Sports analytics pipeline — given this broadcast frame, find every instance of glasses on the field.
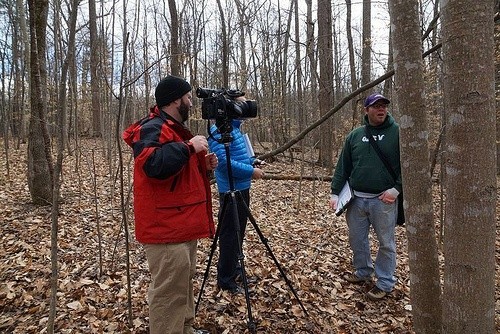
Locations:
[369,104,387,108]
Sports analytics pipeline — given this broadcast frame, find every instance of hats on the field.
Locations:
[364,93,390,108]
[155,75,191,107]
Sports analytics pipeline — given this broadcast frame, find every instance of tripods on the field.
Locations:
[195,134,310,334]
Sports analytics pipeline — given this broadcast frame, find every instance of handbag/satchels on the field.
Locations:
[397,193,405,226]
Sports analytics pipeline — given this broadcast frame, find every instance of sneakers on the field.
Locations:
[343,274,372,283]
[367,286,387,300]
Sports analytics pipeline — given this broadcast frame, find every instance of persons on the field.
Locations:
[209,88,265,296]
[123,75,218,334]
[330,94,405,300]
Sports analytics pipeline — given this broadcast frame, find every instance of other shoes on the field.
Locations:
[217,273,243,296]
